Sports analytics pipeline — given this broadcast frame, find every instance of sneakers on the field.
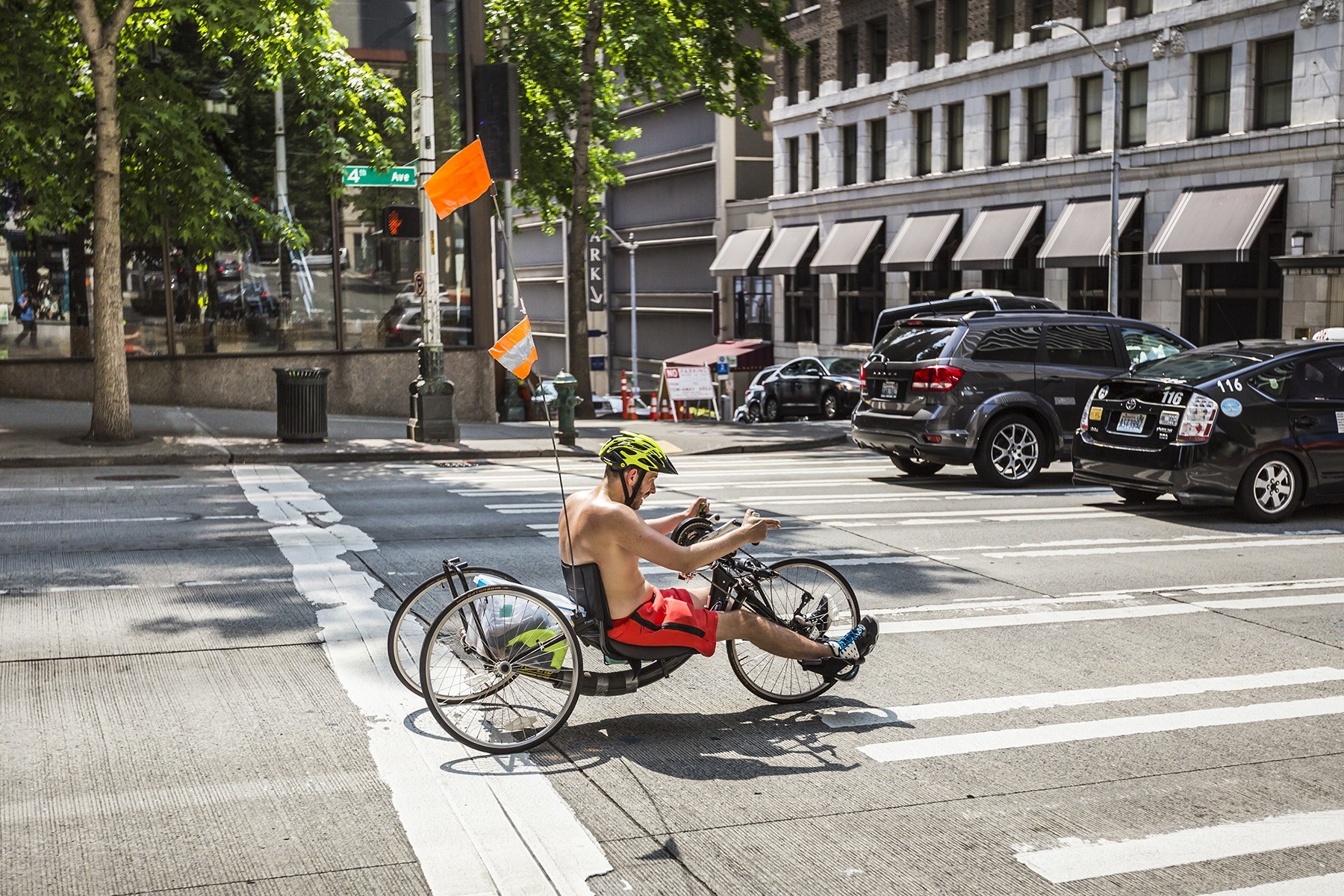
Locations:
[806,594,833,641]
[826,614,879,675]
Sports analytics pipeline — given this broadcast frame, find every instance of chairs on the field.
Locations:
[562,559,632,662]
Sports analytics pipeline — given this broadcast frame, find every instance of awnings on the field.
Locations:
[879,212,960,271]
[951,205,1044,270]
[1148,184,1285,266]
[757,225,819,275]
[1035,194,1143,268]
[810,219,884,275]
[709,228,772,276]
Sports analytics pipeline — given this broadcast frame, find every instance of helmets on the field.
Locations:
[599,431,678,474]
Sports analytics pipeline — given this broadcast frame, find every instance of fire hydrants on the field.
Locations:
[550,369,584,446]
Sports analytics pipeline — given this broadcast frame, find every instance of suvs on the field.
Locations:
[871,287,1071,352]
[845,310,1241,490]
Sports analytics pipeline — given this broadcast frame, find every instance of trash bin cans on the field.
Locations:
[271,366,332,441]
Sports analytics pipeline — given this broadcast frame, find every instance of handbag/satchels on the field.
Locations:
[11,297,22,318]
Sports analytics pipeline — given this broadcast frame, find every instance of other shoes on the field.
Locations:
[30,346,38,349]
[15,341,20,347]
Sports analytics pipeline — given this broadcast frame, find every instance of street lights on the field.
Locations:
[603,224,640,396]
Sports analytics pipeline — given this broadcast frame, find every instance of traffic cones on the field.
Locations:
[647,391,660,420]
[661,398,670,420]
[627,392,639,420]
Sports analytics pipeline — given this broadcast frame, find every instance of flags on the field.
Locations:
[422,138,492,220]
[487,313,540,381]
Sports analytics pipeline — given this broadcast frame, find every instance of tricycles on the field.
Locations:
[386,496,863,756]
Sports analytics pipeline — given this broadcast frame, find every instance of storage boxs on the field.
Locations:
[465,575,586,673]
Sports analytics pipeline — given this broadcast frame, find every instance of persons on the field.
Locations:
[558,429,880,682]
[1135,335,1160,363]
[14,288,40,348]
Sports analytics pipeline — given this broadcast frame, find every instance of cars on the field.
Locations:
[130,255,280,330]
[531,378,651,419]
[291,246,349,272]
[1069,336,1344,524]
[374,302,475,347]
[730,354,865,424]
[393,282,451,308]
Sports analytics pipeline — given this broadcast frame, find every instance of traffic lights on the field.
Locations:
[382,205,421,241]
